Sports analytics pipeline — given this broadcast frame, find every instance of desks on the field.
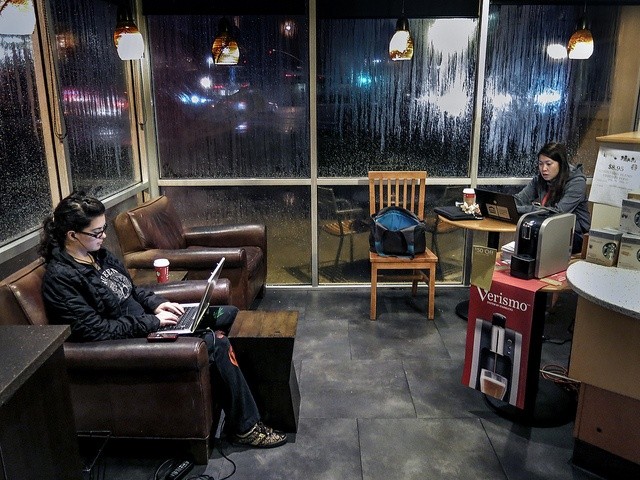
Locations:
[565,261,639,479]
[470,253,578,428]
[0,324,81,480]
[438,207,517,321]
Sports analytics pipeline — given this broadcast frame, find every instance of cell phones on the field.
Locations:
[149,333,179,342]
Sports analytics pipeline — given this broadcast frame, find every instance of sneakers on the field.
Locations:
[230,423,289,449]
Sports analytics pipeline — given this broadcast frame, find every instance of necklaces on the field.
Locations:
[73,253,94,264]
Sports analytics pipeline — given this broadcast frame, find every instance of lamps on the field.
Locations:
[113,8,145,60]
[389,1,414,61]
[212,7,239,65]
[568,0,595,60]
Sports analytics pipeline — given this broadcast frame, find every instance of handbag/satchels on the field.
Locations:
[369,206,426,259]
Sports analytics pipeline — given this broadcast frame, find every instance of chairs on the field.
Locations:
[317,186,369,271]
[368,171,439,320]
[424,187,469,282]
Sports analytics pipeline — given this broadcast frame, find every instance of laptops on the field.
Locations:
[433,204,483,221]
[150,255,227,336]
[475,188,522,224]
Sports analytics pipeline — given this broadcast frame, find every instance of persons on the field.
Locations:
[36,194,289,449]
[512,143,590,255]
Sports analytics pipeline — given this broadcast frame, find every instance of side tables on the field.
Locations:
[128,268,188,284]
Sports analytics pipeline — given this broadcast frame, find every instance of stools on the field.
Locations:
[227,310,302,434]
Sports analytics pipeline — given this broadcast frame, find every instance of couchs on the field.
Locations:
[0,257,232,430]
[112,194,267,311]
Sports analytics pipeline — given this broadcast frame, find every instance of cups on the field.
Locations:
[462,188,475,205]
[153,258,170,282]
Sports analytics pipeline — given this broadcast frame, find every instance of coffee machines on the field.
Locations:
[468,312,523,407]
[509,201,577,281]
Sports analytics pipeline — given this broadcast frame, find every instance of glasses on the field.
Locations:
[77,224,108,238]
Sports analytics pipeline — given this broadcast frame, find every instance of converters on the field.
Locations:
[165,458,193,479]
